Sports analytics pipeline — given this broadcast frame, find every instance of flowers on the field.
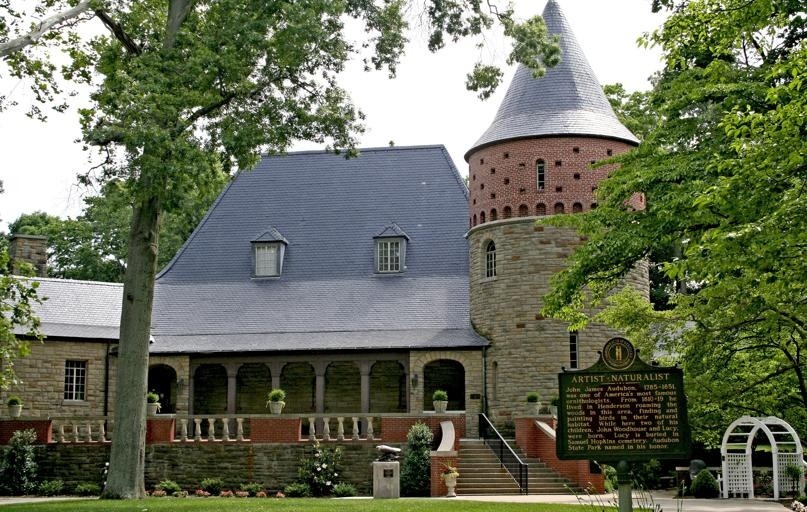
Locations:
[439,458,460,474]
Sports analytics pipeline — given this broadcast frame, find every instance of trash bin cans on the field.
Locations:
[372,461,400,499]
[675,467,690,490]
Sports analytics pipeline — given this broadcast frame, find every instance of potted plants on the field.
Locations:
[265,389,285,414]
[432,390,448,413]
[146,392,161,417]
[5,396,23,419]
[525,391,558,416]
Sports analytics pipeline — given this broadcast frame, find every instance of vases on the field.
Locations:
[443,473,458,497]
